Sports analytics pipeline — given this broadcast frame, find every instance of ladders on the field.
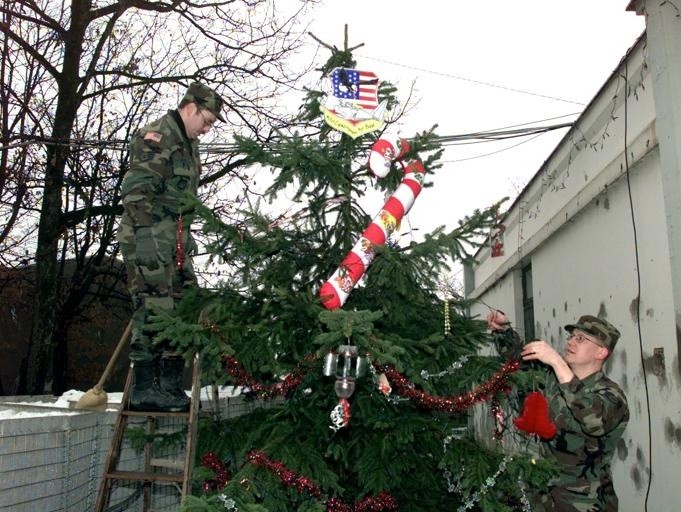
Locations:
[94,353,201,512]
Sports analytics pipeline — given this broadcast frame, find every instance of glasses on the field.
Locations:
[566,333,604,348]
[199,110,213,129]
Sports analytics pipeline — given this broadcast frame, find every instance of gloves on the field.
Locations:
[135,227,166,271]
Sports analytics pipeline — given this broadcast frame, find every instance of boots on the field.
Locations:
[128,355,202,413]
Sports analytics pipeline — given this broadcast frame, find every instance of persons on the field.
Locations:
[115,81,227,414]
[486,308,630,511]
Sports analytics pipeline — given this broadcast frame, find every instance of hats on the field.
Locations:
[564,315,621,352]
[184,81,226,123]
